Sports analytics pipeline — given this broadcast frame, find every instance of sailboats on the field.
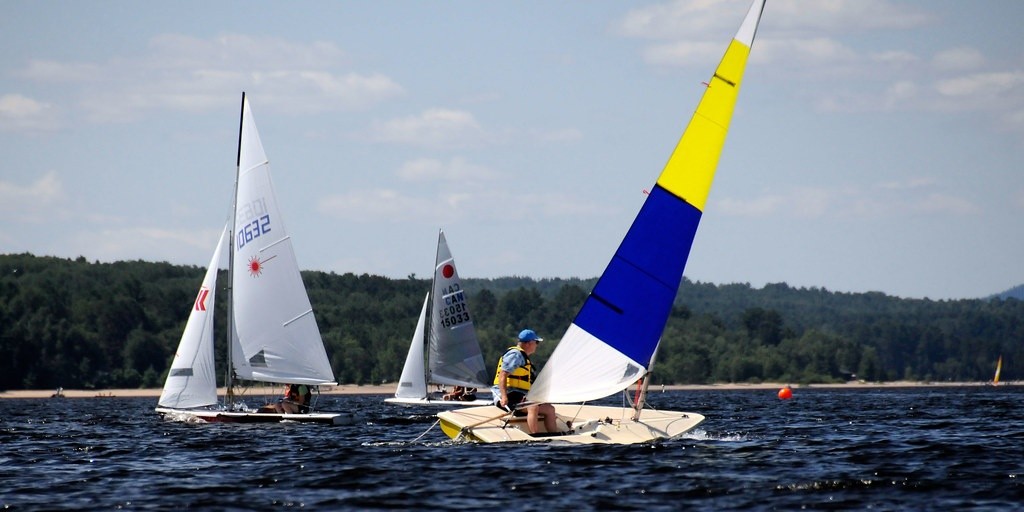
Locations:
[380,228,495,409]
[437,1,766,444]
[154,92,353,428]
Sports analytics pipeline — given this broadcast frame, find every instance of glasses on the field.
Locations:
[531,341,539,344]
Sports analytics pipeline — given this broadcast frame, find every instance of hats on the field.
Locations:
[518,329,543,342]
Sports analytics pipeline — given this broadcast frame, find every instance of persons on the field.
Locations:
[275,384,311,413]
[492,330,556,433]
[443,386,477,401]
